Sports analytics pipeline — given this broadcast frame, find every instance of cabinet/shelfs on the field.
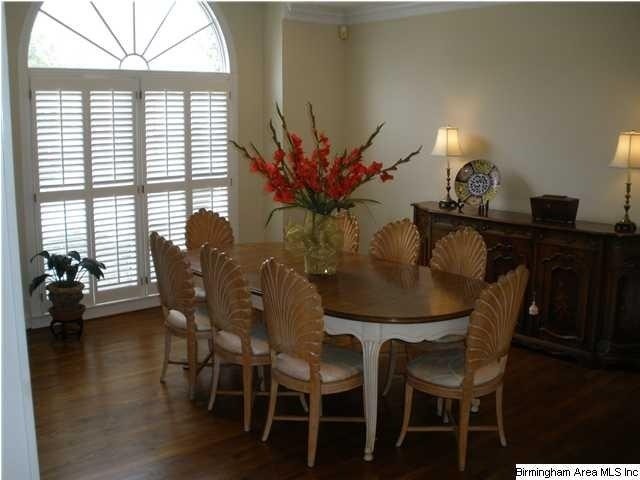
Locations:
[412,205,640,371]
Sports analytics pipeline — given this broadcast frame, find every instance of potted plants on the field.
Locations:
[29,251,107,321]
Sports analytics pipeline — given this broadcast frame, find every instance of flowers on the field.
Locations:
[225,101,424,229]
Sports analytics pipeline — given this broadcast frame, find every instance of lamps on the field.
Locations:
[430,123,467,210]
[606,130,640,234]
